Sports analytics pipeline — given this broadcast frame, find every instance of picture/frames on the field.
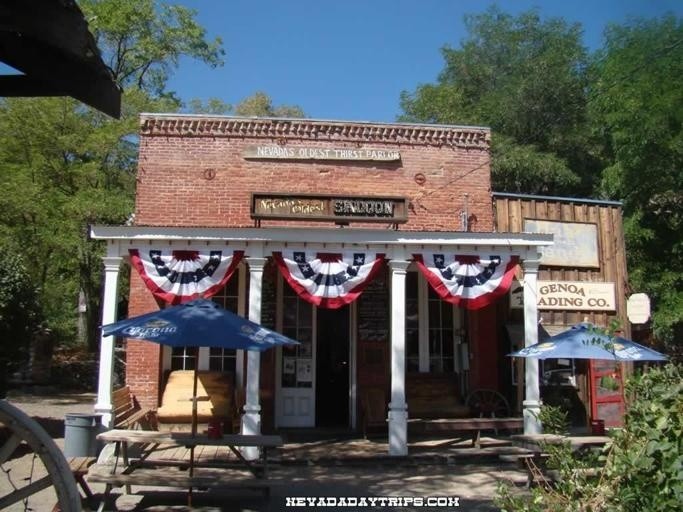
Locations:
[523,217,601,271]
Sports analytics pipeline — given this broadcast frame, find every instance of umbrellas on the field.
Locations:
[505,318,670,439]
[98,291,302,507]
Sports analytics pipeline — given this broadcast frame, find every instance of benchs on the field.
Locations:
[53,453,97,505]
[357,371,473,442]
[485,446,541,486]
[125,456,284,479]
[86,474,285,512]
[110,384,152,431]
[422,418,524,448]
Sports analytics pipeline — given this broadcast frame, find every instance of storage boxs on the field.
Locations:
[94,429,282,480]
